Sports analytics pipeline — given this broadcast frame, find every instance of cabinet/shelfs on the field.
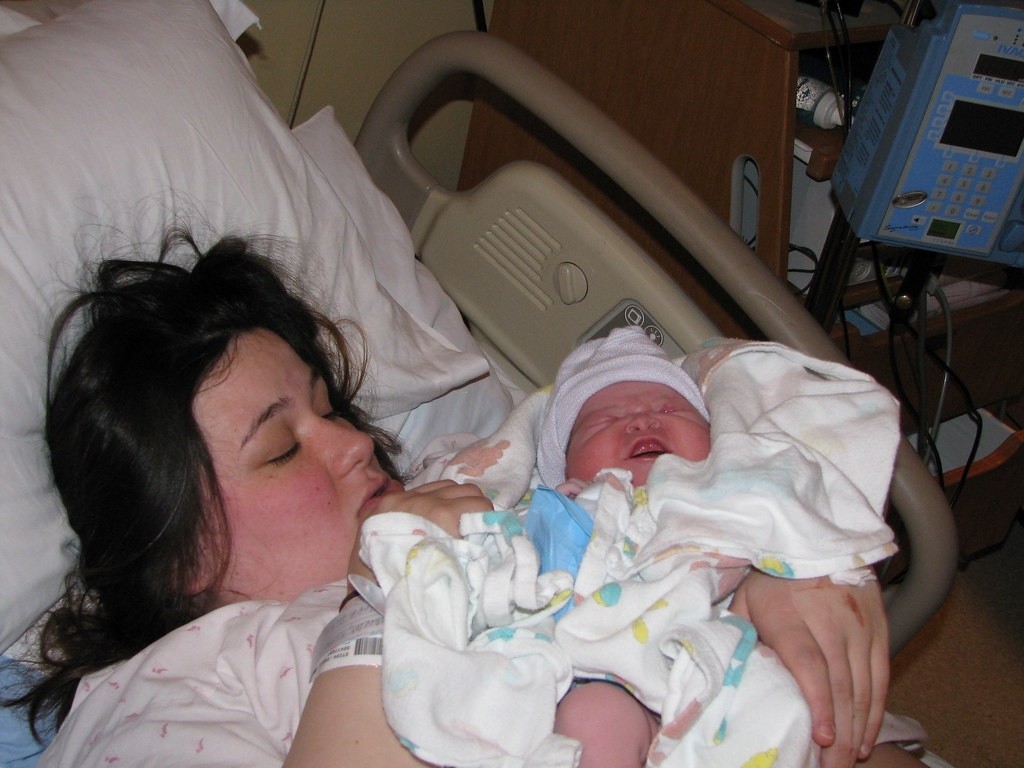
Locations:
[457,0,1024,588]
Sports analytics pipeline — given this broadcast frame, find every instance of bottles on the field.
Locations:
[795,75,857,131]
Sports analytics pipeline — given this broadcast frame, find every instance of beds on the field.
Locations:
[0,0,958,768]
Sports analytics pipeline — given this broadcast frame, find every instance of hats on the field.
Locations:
[535,325,710,489]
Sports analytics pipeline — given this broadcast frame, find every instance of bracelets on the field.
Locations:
[308,591,384,684]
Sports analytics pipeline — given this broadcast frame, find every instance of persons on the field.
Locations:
[518,327,933,768]
[0,229,893,768]
